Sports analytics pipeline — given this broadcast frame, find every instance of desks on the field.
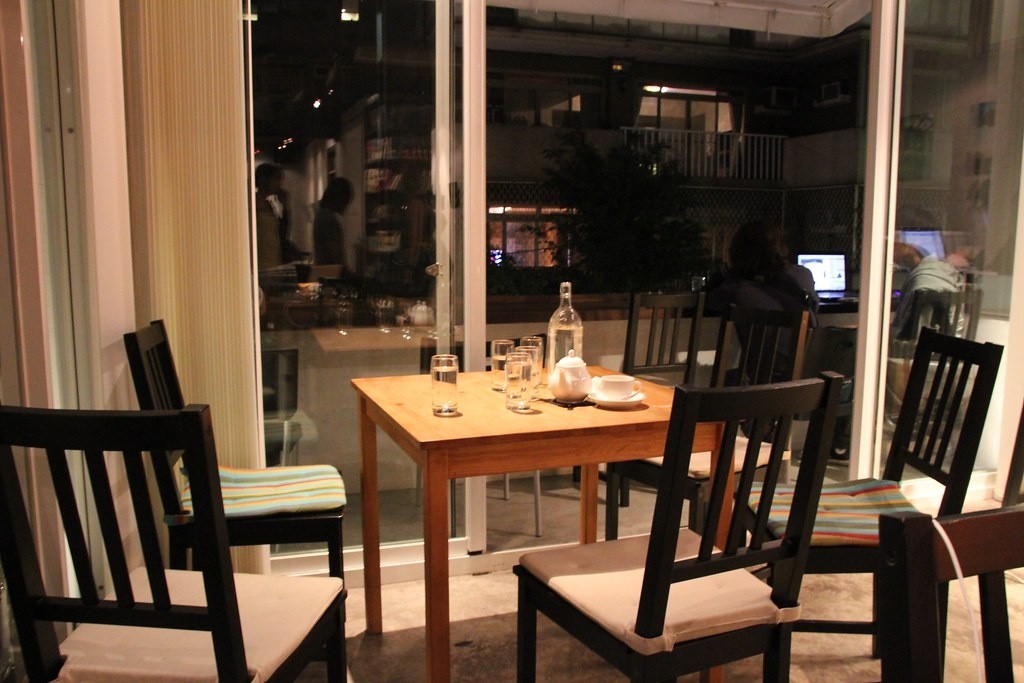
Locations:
[351,364,733,683]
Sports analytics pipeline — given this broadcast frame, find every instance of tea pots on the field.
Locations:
[548,349,591,404]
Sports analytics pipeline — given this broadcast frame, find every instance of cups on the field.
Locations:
[505,352,532,411]
[491,339,514,392]
[430,355,457,416]
[516,346,541,402]
[522,337,543,387]
[601,375,644,402]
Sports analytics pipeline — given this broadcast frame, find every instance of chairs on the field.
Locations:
[0,403,349,683]
[725,326,1003,678]
[902,290,984,361]
[877,508,1024,683]
[571,270,819,538]
[122,319,349,663]
[513,371,843,683]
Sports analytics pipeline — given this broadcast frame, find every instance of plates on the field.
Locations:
[589,390,647,409]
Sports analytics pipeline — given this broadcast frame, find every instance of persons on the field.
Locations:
[312,176,354,264]
[797,242,965,463]
[407,206,436,300]
[627,218,819,444]
[343,200,421,327]
[255,160,291,309]
[941,195,991,270]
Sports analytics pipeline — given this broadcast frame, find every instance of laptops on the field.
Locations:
[890,227,946,298]
[796,253,848,300]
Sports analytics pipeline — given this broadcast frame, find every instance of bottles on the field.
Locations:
[547,282,583,374]
